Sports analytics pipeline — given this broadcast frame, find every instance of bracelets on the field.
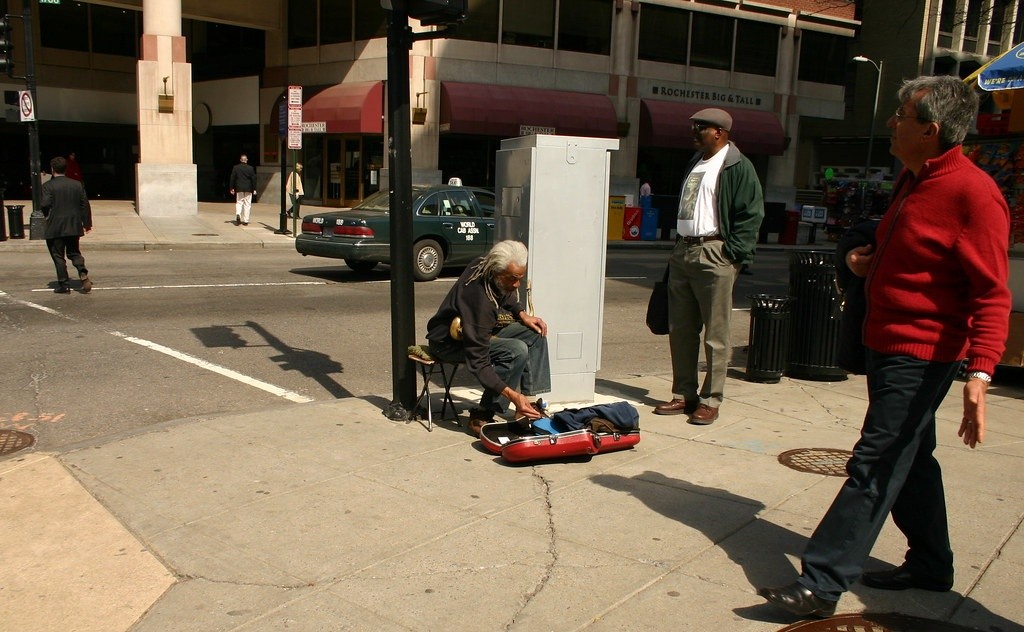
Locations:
[970,372,992,386]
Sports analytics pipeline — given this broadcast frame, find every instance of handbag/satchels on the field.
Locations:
[834,282,867,374]
[646,261,669,335]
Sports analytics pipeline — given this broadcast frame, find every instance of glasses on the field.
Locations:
[691,123,717,130]
[895,108,934,123]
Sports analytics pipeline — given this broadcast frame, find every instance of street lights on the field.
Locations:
[852,55,884,178]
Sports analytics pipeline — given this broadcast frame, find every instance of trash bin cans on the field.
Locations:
[4,203,26,239]
[743,290,790,385]
[789,252,853,382]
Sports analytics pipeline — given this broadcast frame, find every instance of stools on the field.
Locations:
[401,345,472,432]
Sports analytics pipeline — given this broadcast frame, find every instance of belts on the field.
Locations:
[677,235,720,243]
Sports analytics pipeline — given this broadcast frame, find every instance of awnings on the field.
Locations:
[439,80,619,140]
[269,80,384,134]
[641,98,792,158]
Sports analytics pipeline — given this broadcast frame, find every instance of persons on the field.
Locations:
[757,76,1007,618]
[230,155,257,226]
[654,108,763,425]
[286,163,304,219]
[425,240,551,438]
[66,151,82,182]
[41,157,92,294]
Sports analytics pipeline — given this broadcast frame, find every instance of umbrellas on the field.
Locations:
[962,39,1024,90]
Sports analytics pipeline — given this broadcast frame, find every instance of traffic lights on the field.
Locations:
[0,17,17,75]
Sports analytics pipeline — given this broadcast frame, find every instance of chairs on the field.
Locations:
[453,206,467,216]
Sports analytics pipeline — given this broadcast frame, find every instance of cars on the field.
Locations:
[294,177,495,281]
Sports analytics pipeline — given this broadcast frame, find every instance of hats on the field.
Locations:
[689,108,732,132]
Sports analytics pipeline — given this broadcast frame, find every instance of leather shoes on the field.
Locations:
[655,395,700,415]
[691,404,719,424]
[862,561,951,592]
[758,582,837,618]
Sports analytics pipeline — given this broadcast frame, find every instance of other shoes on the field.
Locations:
[81,273,91,292]
[55,287,69,293]
[514,402,546,420]
[237,215,240,223]
[243,222,247,225]
[287,211,291,217]
[466,416,492,438]
